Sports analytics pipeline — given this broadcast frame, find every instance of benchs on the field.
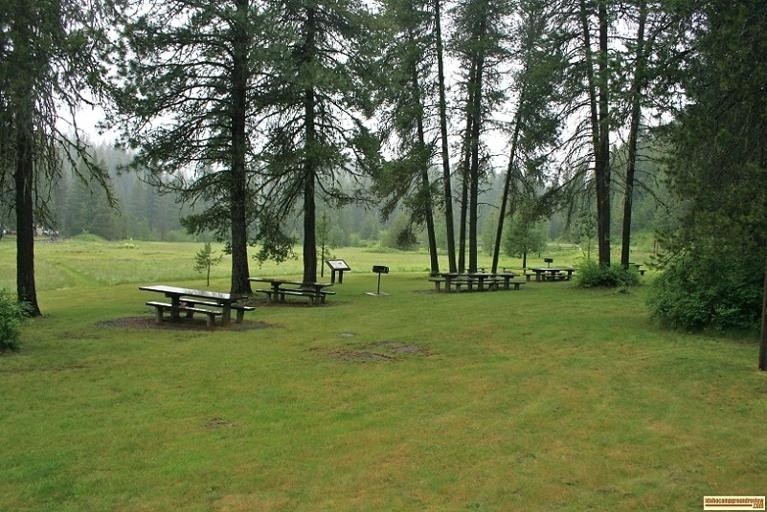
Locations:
[255,287,336,305]
[144,298,255,328]
[525,273,575,282]
[427,278,526,293]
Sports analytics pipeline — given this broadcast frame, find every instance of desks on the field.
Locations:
[528,266,575,282]
[139,284,248,326]
[436,272,520,293]
[248,277,332,306]
